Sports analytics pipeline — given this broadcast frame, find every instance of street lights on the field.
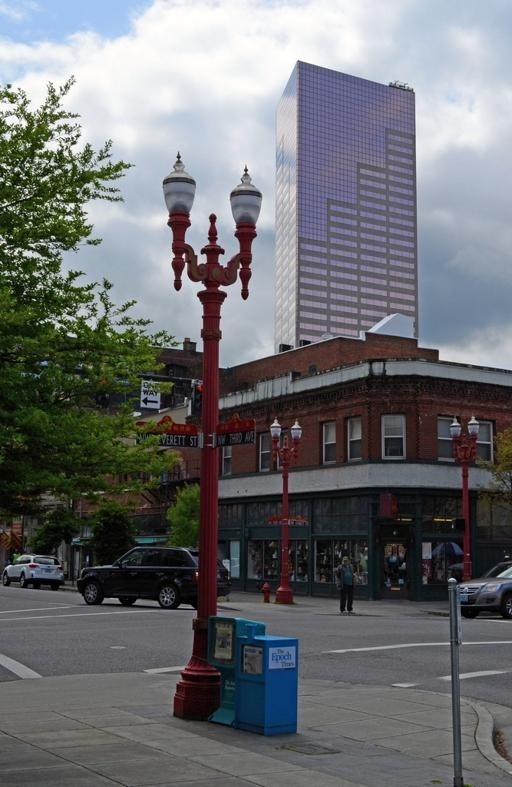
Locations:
[270,416,303,604]
[162,150,262,722]
[449,414,479,581]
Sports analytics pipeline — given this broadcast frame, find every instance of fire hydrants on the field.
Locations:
[262,582,271,602]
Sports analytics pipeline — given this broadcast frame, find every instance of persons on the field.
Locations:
[333,556,355,612]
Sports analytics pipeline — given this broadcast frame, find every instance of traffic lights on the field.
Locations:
[192,383,204,418]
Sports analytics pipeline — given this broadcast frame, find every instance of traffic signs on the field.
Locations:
[140,379,161,409]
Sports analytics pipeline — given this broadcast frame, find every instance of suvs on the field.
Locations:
[76,546,232,608]
[457,560,512,618]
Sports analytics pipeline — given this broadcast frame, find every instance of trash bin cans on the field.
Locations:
[210,615,266,727]
[239,636,296,736]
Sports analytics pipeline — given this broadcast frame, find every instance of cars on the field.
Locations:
[2,553,65,590]
